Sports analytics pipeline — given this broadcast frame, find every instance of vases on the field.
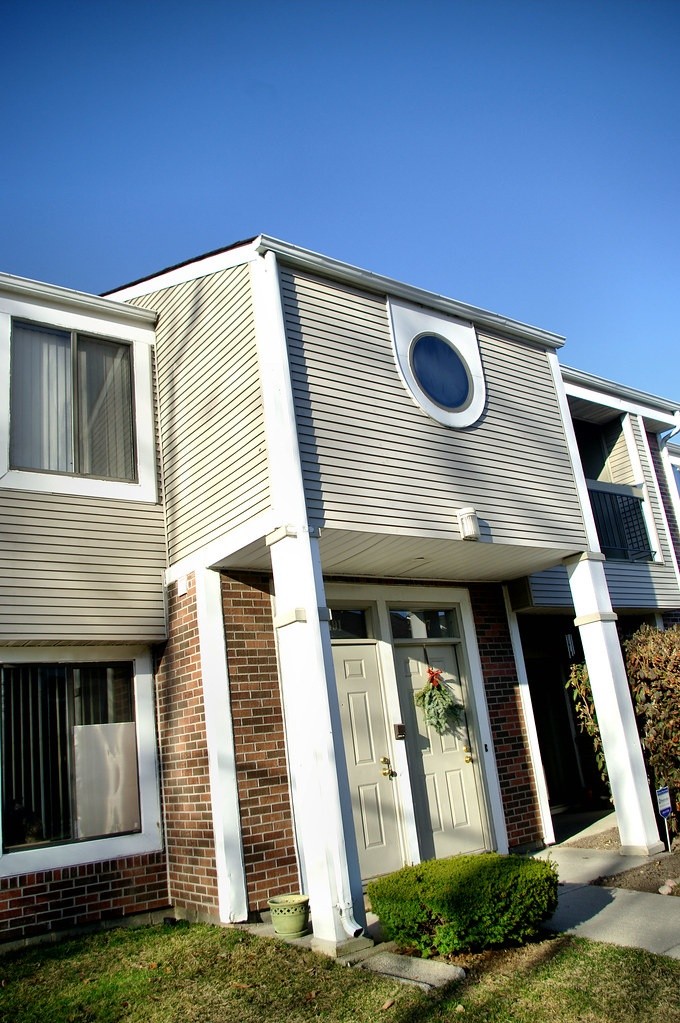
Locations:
[268,895,309,939]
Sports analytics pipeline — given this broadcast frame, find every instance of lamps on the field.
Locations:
[457,507,481,540]
